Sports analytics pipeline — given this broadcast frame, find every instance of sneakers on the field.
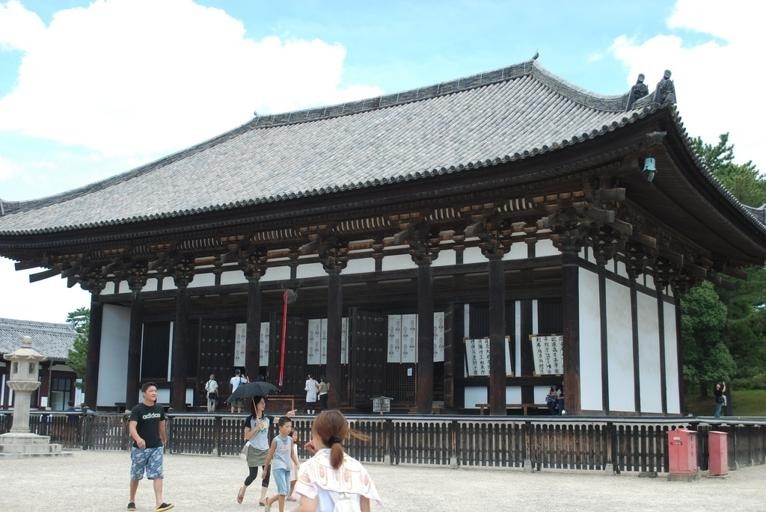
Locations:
[127,502,137,511]
[259,496,297,512]
[156,502,174,512]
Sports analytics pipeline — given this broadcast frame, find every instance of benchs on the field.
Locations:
[475,403,547,416]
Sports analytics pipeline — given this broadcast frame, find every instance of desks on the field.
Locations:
[265,394,306,411]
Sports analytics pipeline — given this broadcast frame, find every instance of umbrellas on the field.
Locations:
[225,381,280,403]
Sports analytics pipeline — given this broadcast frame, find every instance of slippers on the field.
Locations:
[237,485,245,504]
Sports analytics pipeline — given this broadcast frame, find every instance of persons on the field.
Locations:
[286,427,299,502]
[78,401,95,419]
[548,384,558,415]
[319,376,330,409]
[714,381,727,417]
[235,394,298,506]
[229,368,248,413]
[558,378,565,414]
[203,374,219,412]
[65,400,79,421]
[290,410,372,512]
[126,381,174,511]
[262,415,298,512]
[304,370,320,415]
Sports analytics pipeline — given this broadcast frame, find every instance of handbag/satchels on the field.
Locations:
[208,390,219,399]
[239,440,251,460]
